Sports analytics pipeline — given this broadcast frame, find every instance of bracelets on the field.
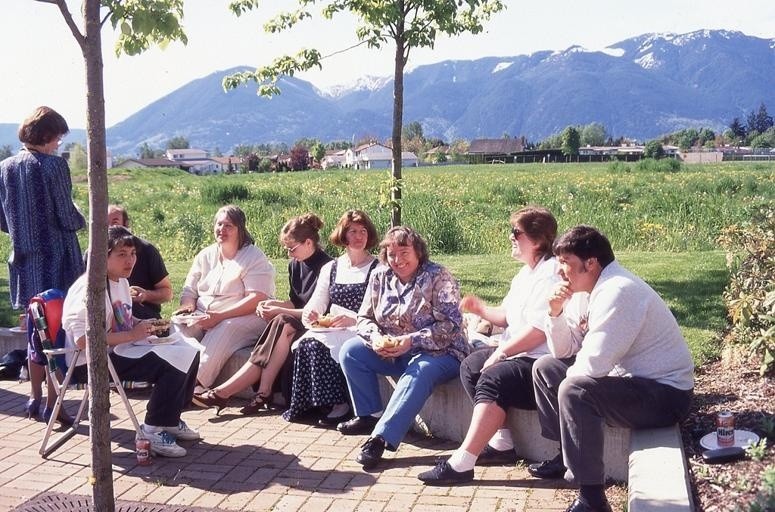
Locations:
[494,349,508,360]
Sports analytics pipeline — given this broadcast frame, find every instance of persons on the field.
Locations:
[81,203,173,320]
[283,210,380,425]
[528,226,694,512]
[0,105,85,427]
[335,226,470,468]
[191,214,334,415]
[61,225,200,456]
[169,204,277,396]
[417,206,591,485]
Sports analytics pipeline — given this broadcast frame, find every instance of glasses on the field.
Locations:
[56,135,64,146]
[511,227,526,239]
[284,243,303,253]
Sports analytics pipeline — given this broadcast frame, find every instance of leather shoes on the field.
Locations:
[567,494,613,512]
[528,453,566,479]
[476,443,517,466]
[418,460,474,485]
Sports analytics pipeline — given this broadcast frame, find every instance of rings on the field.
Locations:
[147,327,152,333]
[553,290,558,296]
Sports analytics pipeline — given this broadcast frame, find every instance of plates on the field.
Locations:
[699,429,759,450]
[310,327,345,332]
[173,315,206,320]
[133,337,177,346]
[9,326,28,333]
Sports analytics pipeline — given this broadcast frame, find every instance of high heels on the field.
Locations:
[24,400,40,420]
[240,392,273,413]
[192,390,227,415]
[43,407,75,425]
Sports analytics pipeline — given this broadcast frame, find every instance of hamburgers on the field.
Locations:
[377,332,398,359]
[311,312,334,327]
[146,325,177,344]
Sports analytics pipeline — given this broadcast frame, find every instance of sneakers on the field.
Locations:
[319,406,352,426]
[356,437,385,465]
[135,425,187,458]
[337,415,380,435]
[162,419,199,441]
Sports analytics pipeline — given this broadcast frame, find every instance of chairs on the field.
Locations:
[30,299,149,455]
